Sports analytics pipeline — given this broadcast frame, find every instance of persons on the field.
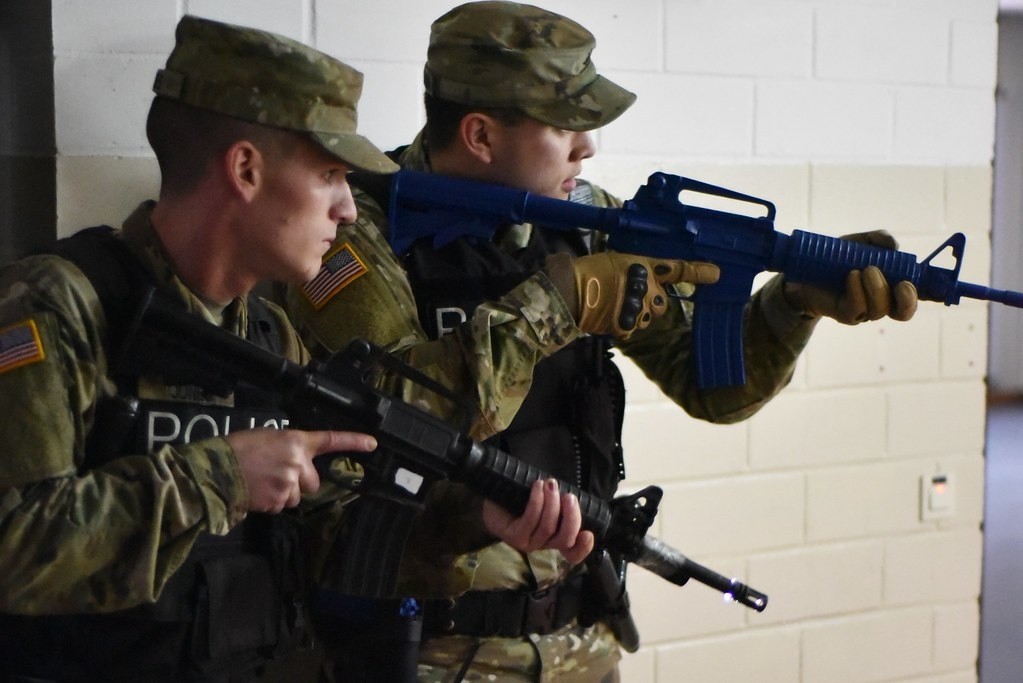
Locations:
[0,13,594,683]
[274,0,918,683]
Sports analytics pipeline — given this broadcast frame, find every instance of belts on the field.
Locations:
[411,566,585,638]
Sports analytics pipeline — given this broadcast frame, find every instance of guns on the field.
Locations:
[347,172,1023,389]
[84,280,770,614]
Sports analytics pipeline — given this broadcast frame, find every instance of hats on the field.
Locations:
[424,0,638,132]
[151,14,401,175]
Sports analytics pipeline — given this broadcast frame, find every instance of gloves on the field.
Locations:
[532,248,720,342]
[783,228,918,326]
